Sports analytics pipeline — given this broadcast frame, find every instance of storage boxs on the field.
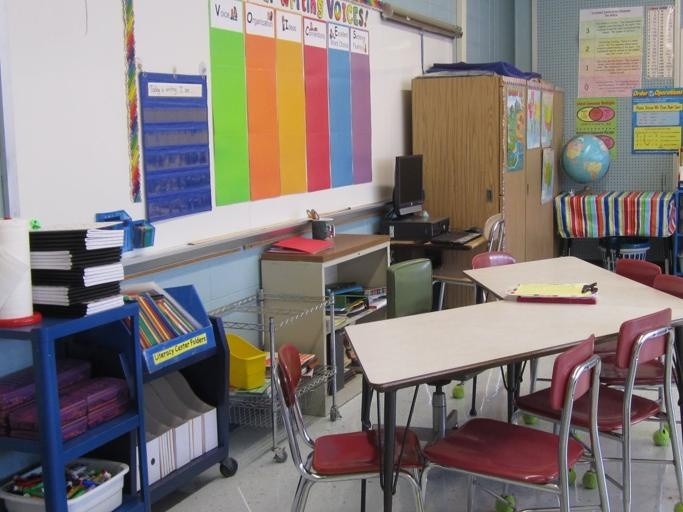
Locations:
[0,456,129,511]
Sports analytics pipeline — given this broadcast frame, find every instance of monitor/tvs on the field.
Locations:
[392,154,424,215]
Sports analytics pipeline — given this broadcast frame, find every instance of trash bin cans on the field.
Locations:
[597,236,654,273]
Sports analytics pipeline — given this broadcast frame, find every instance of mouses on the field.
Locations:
[465,226,481,233]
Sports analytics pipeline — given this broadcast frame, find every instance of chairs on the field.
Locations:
[434,211,505,314]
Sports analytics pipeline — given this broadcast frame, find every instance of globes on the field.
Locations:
[561,134,611,195]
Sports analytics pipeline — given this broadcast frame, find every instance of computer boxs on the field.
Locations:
[383,216,450,240]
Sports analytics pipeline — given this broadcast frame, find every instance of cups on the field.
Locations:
[311,217,337,242]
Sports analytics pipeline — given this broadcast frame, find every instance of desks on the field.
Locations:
[376,219,485,267]
[551,189,673,276]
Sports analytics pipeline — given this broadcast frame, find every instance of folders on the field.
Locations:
[325,294,368,309]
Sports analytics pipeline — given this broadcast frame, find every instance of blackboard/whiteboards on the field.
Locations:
[0,0,490,278]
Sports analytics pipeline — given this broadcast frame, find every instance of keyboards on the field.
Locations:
[431,229,481,246]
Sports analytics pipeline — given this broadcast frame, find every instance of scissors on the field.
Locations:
[307,210,319,220]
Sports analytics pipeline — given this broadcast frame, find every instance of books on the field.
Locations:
[28,227,126,316]
[226,350,321,399]
[116,280,201,348]
[364,287,391,310]
[265,236,336,255]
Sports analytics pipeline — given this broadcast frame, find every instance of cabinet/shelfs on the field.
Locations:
[412,70,526,282]
[0,296,138,512]
[211,288,341,464]
[113,282,238,512]
[260,235,393,417]
[525,78,567,260]
[669,152,683,278]
